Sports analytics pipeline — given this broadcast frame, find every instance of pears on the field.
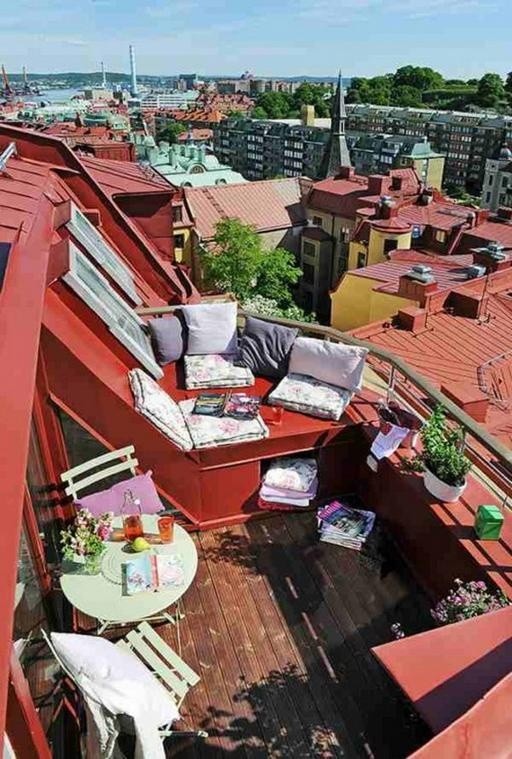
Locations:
[132,534,150,552]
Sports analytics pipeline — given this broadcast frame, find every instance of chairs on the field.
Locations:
[41,621,208,742]
[60,445,139,501]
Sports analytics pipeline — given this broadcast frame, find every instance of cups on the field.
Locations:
[157,512,175,543]
[270,393,285,425]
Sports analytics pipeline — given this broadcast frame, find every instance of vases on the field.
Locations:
[82,555,102,575]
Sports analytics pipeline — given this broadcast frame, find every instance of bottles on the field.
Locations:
[386,388,403,424]
[378,399,400,426]
[120,488,144,543]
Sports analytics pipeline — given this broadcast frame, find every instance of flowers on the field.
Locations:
[59,508,114,565]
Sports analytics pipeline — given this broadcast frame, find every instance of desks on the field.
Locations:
[59,513,198,635]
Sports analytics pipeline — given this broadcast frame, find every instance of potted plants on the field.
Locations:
[399,405,468,503]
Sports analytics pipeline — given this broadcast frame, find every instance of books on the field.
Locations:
[191,389,262,420]
[316,500,377,552]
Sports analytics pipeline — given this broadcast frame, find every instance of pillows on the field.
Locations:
[73,470,165,520]
[50,631,182,728]
[232,314,298,374]
[148,315,183,364]
[288,336,369,392]
[182,301,238,355]
